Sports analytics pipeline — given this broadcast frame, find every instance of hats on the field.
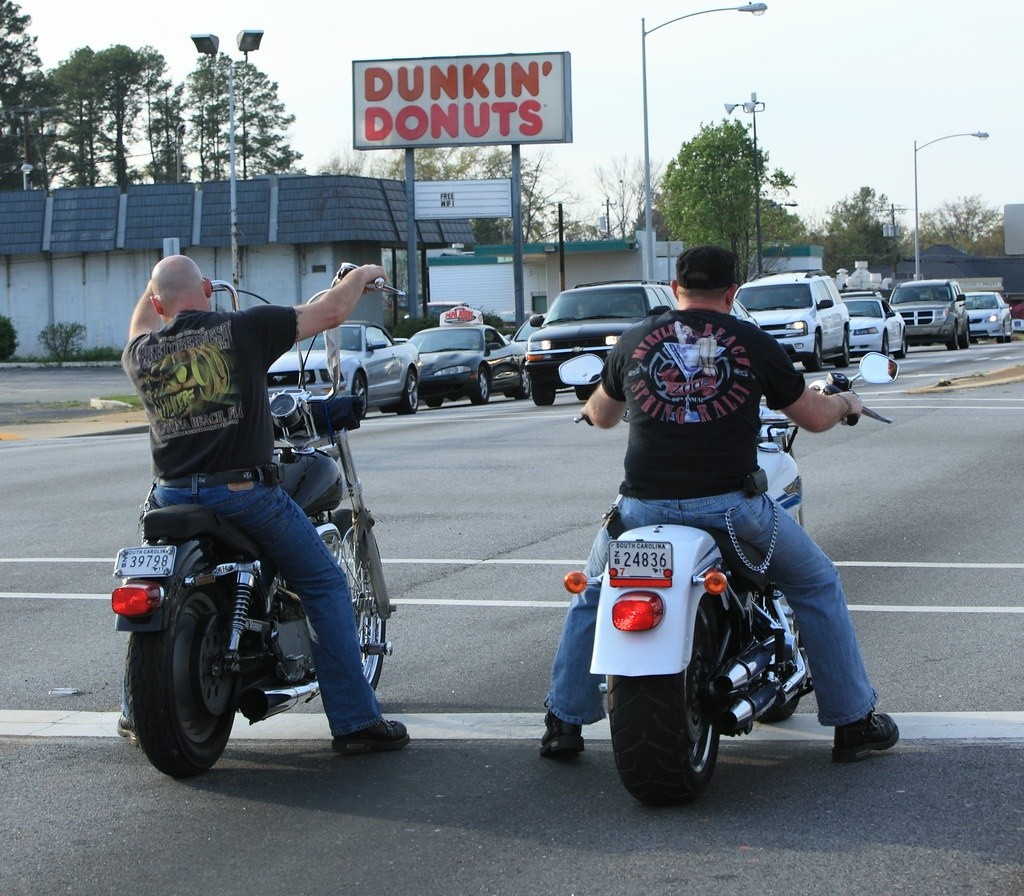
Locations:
[675,246,737,289]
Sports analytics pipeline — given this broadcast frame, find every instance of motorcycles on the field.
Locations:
[560,344,901,808]
[113,259,408,779]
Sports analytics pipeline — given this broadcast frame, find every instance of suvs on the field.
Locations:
[526,279,678,406]
[733,269,852,372]
[883,278,971,350]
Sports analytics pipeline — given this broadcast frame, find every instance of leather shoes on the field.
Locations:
[332,720,411,754]
[833,713,900,760]
[118,717,139,740]
[539,710,585,757]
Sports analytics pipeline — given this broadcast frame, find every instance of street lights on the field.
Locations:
[641,0,767,281]
[722,92,764,277]
[191,29,265,290]
[914,131,989,278]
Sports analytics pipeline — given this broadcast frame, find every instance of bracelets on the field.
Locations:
[837,394,852,420]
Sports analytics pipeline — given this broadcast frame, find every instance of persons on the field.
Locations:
[116,255,411,752]
[625,298,640,313]
[541,245,900,761]
[468,332,480,346]
[578,301,588,317]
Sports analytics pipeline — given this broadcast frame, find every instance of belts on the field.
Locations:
[154,468,257,486]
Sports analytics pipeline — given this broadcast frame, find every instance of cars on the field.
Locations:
[1010,301,1024,332]
[379,305,531,414]
[823,290,910,365]
[403,300,469,322]
[728,298,761,329]
[504,312,550,399]
[265,319,420,437]
[962,291,1015,344]
